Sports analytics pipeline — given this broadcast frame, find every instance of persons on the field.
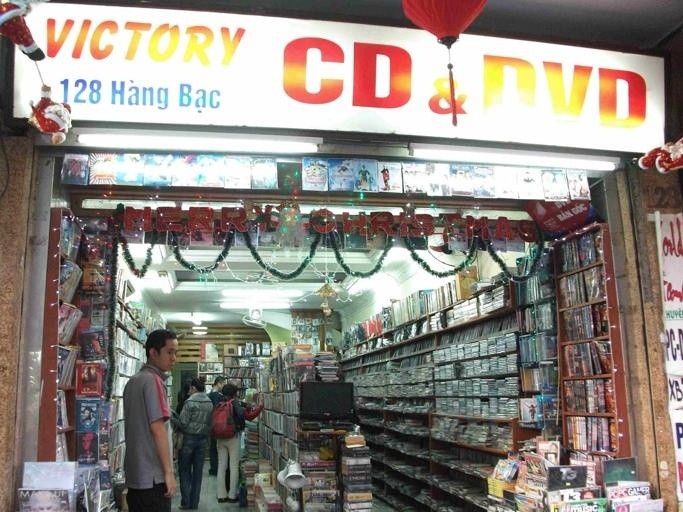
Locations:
[123,329,178,512]
[176,377,213,510]
[208,377,225,475]
[214,384,264,503]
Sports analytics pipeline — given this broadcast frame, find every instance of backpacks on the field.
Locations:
[210,397,245,438]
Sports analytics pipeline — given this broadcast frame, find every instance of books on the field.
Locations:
[514,451,546,507]
[600,457,664,512]
[546,465,606,512]
[340,434,373,512]
[20,462,77,508]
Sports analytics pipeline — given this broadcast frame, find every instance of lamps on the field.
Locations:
[314,284,338,297]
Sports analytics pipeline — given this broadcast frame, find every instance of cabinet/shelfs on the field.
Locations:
[197,361,223,385]
[515,248,562,424]
[340,276,542,512]
[222,355,274,402]
[547,221,632,458]
[37,207,77,462]
[109,295,173,484]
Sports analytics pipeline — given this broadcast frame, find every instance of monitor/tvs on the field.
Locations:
[300,381,354,426]
